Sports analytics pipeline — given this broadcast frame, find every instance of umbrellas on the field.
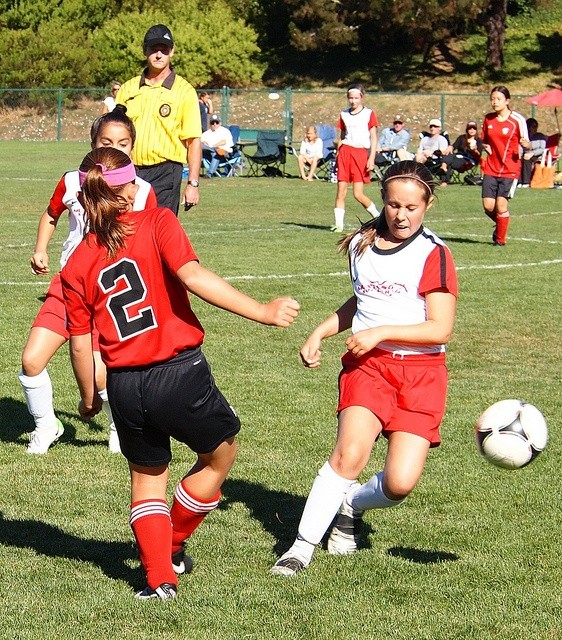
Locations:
[523,88,562,135]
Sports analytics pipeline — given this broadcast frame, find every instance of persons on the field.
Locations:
[17,103,123,457]
[101,79,122,116]
[297,125,323,180]
[199,91,213,134]
[439,121,482,188]
[59,147,299,600]
[479,85,531,247]
[375,114,411,164]
[517,117,547,189]
[200,114,235,178]
[329,85,381,233]
[114,24,203,219]
[415,118,448,163]
[266,161,459,578]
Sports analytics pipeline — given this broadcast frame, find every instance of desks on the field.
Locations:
[236,141,257,176]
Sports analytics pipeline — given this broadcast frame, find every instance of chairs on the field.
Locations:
[245,131,285,178]
[202,125,240,177]
[545,133,560,185]
[299,125,336,180]
[419,131,451,185]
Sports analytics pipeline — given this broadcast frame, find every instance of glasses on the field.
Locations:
[467,128,478,130]
[532,125,538,128]
[430,126,439,128]
[113,89,119,90]
[210,121,219,125]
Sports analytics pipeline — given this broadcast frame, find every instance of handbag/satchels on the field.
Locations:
[530,149,557,189]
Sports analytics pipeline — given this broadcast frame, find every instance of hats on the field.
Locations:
[144,24,173,47]
[467,122,477,127]
[210,114,221,122]
[393,114,405,123]
[429,118,441,127]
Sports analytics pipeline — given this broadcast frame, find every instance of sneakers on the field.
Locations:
[270,533,318,577]
[492,225,496,240]
[172,545,194,574]
[328,482,363,556]
[331,224,341,233]
[109,420,121,453]
[517,184,522,188]
[522,184,529,188]
[439,183,447,187]
[439,166,447,174]
[494,241,505,246]
[133,582,177,600]
[25,417,65,454]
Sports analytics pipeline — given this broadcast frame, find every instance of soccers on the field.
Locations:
[476,399,550,468]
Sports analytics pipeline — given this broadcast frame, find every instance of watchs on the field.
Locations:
[186,179,201,188]
